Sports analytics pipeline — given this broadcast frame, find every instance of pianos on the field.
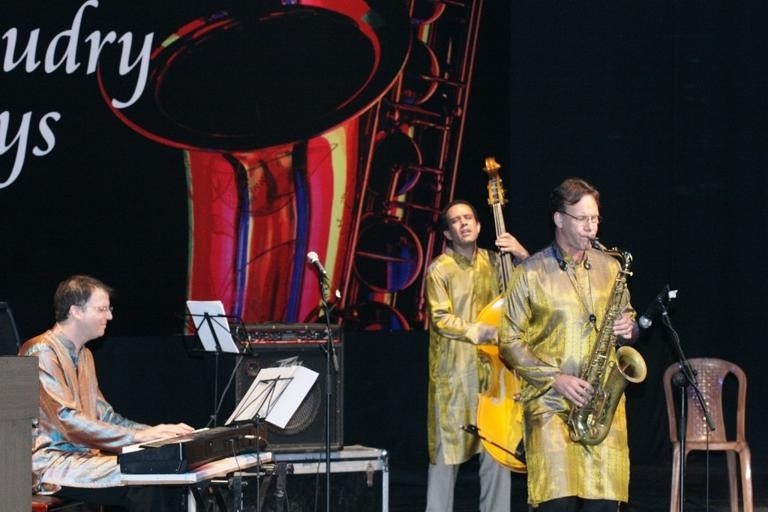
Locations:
[117,419,266,473]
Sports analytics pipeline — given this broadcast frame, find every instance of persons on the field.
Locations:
[424,200,529,512]
[15,275,196,512]
[498,177,639,512]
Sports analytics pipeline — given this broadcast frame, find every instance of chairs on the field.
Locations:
[664,359,753,512]
[0,301,88,512]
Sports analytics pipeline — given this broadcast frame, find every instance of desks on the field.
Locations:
[187,445,389,512]
[0,355,39,512]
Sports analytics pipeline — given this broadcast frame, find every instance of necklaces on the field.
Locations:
[552,246,597,332]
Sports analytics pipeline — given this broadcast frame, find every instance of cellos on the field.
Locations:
[475,158,529,475]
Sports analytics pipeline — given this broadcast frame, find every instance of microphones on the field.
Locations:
[639,285,671,328]
[306,251,342,298]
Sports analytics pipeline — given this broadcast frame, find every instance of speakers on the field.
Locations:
[234,323,345,453]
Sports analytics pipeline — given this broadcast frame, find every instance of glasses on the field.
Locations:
[75,300,114,315]
[557,208,604,225]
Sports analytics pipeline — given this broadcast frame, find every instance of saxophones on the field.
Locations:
[568,237,646,445]
